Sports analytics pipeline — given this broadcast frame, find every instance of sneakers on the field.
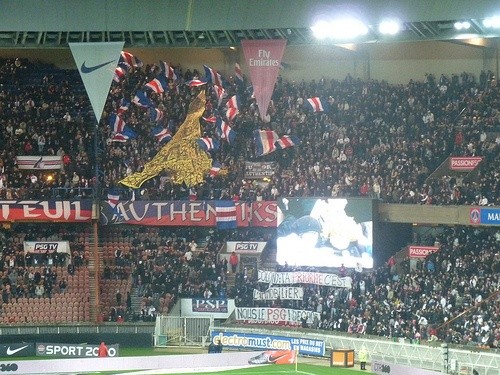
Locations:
[248,351,295,365]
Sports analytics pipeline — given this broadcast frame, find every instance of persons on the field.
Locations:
[0,56,499,353]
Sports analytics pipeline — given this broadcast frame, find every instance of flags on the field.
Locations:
[250,125,297,159]
[107,48,248,152]
[296,95,330,114]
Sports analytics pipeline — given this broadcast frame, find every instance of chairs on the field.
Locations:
[8,68,87,96]
[0,225,171,324]
